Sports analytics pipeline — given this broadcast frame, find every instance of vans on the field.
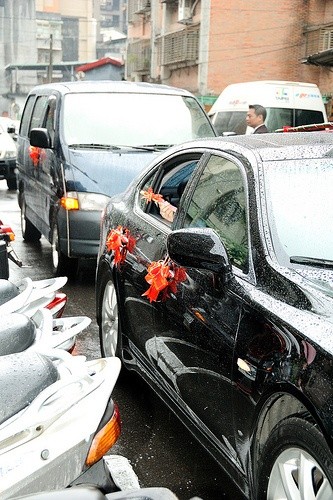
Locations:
[16,79,222,277]
[204,78,330,136]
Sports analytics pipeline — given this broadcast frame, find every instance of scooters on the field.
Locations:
[0,275,200,500]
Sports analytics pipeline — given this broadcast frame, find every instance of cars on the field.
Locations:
[97,133,333,500]
[0,121,21,193]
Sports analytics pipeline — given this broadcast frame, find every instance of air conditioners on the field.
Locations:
[178,6,191,20]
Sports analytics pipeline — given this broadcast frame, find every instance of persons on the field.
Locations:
[245,105,269,134]
